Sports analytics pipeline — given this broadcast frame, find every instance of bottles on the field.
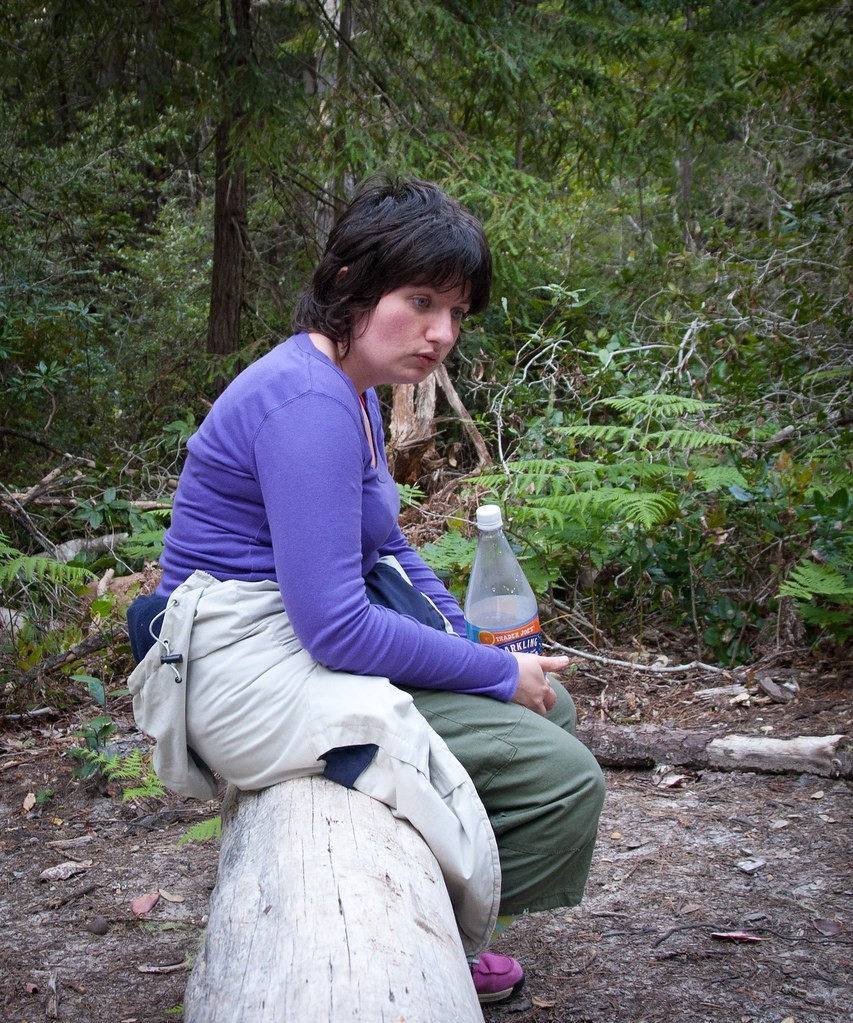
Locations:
[463,505,543,659]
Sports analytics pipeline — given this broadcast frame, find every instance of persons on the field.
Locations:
[128,175,606,1005]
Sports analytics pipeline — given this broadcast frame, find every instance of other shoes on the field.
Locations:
[470,951,525,1004]
